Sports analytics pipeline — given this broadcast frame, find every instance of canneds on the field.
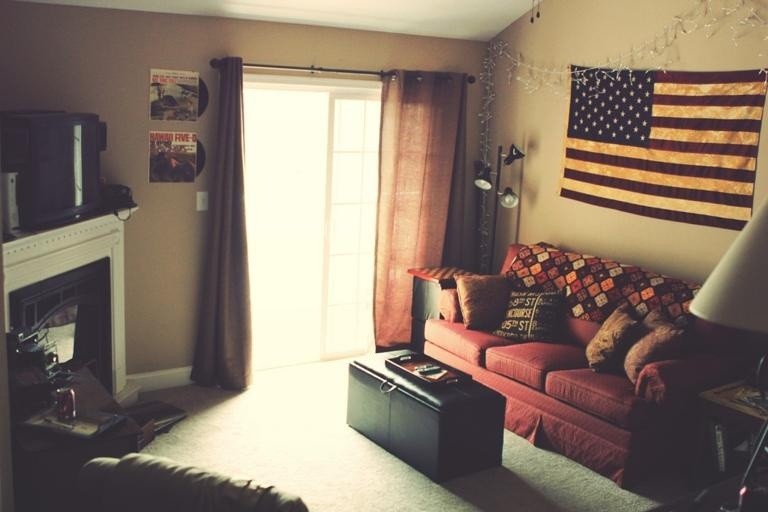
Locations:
[55,388,76,421]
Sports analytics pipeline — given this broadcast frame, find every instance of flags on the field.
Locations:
[558,64,768,230]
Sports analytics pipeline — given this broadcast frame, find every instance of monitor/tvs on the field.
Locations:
[0,114,99,228]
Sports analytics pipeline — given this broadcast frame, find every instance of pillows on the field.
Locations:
[623,310,700,385]
[455,269,517,331]
[491,282,567,345]
[584,298,647,376]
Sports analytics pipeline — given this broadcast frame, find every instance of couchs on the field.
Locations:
[81,453,310,512]
[425,242,725,494]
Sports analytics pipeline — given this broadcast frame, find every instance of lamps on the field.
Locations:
[475,143,527,277]
[692,196,767,508]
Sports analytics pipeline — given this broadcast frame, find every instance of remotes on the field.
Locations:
[417,366,441,374]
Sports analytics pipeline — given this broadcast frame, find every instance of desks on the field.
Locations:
[410,265,483,353]
[699,380,766,492]
[646,494,767,511]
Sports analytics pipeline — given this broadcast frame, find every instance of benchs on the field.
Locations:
[346,348,507,485]
